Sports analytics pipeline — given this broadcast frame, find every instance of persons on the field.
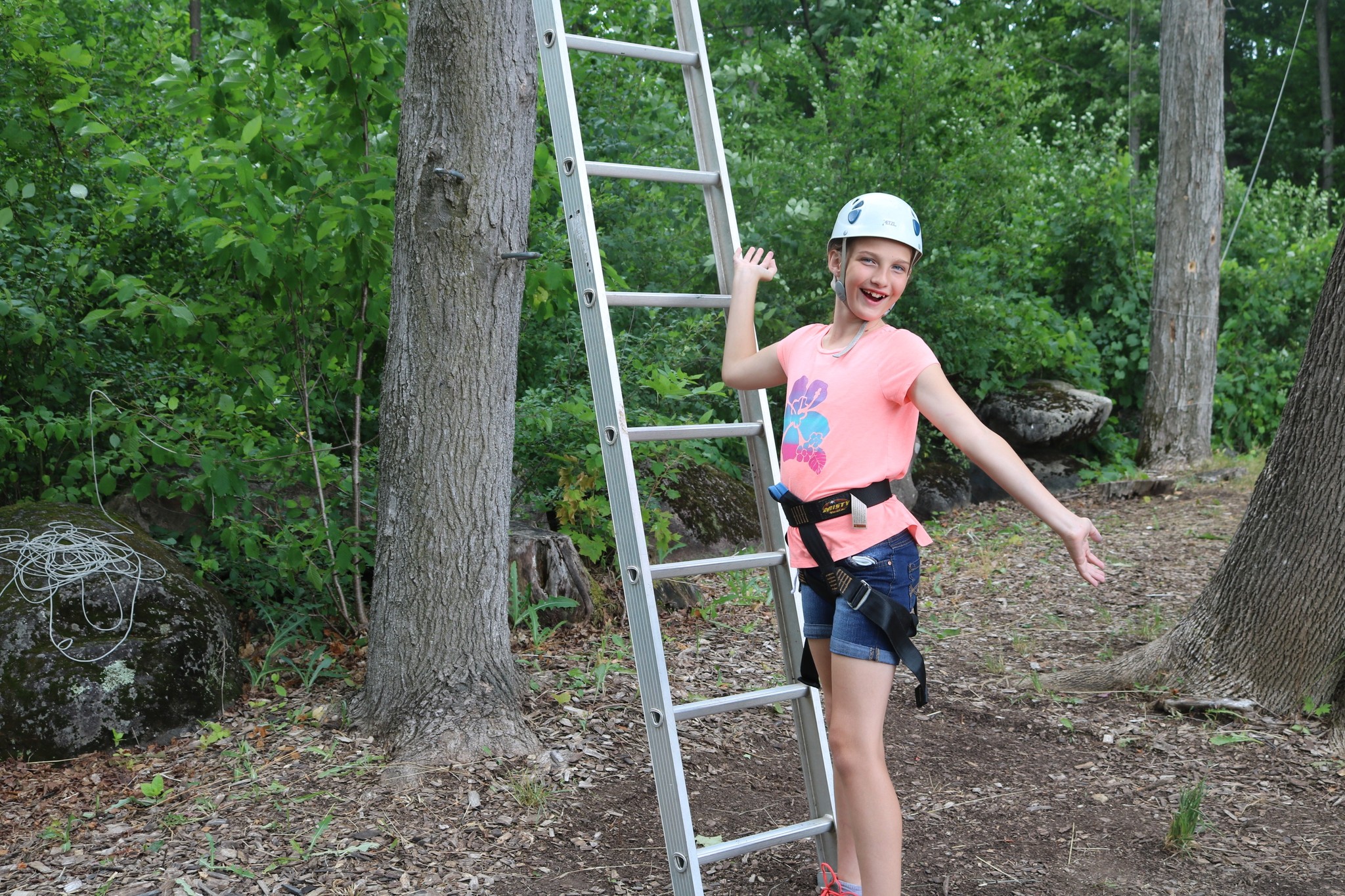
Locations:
[719,193,1106,896]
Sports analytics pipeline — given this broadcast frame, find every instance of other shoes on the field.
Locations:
[818,863,857,896]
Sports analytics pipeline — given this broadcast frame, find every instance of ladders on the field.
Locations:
[538,3,842,892]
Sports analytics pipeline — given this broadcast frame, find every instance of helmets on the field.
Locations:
[826,192,922,266]
